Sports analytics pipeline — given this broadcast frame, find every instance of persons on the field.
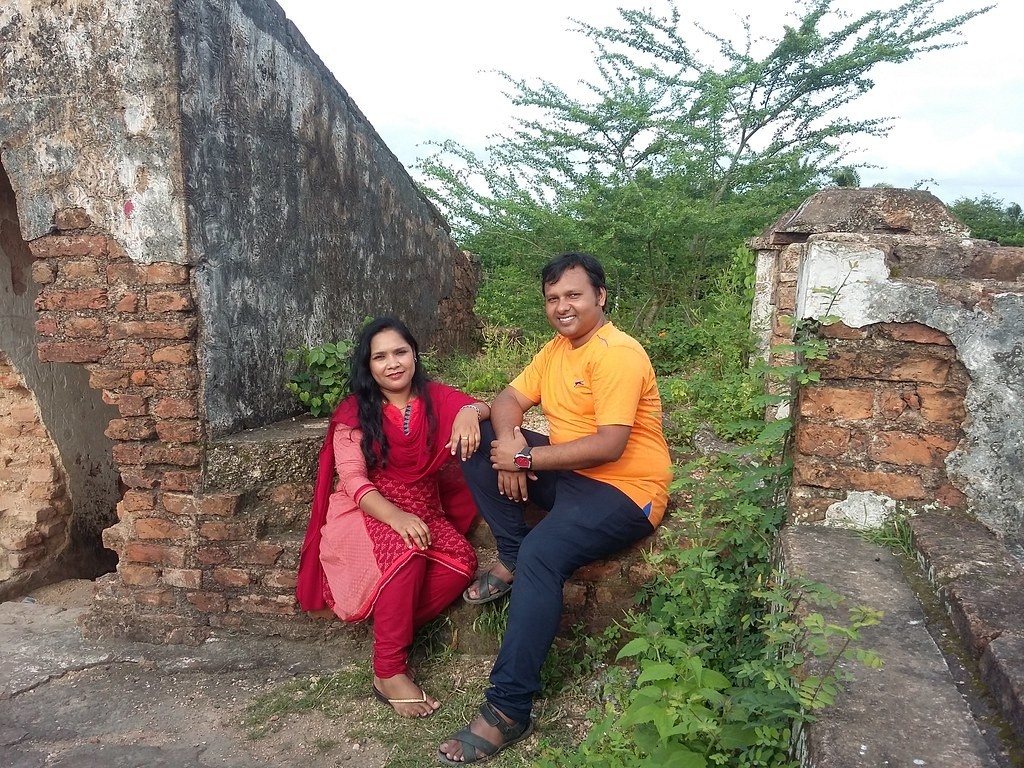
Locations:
[293,317,491,719]
[435,252,674,768]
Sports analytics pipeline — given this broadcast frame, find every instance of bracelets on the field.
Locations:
[457,405,482,420]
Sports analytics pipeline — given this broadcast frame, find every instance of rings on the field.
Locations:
[461,437,468,440]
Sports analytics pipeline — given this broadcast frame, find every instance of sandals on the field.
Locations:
[437,702,533,766]
[462,560,516,605]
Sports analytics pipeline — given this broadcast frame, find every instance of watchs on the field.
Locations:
[512,445,533,471]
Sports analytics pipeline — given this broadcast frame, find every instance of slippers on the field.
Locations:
[373,685,435,719]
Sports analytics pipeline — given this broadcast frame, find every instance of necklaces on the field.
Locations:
[382,392,412,410]
